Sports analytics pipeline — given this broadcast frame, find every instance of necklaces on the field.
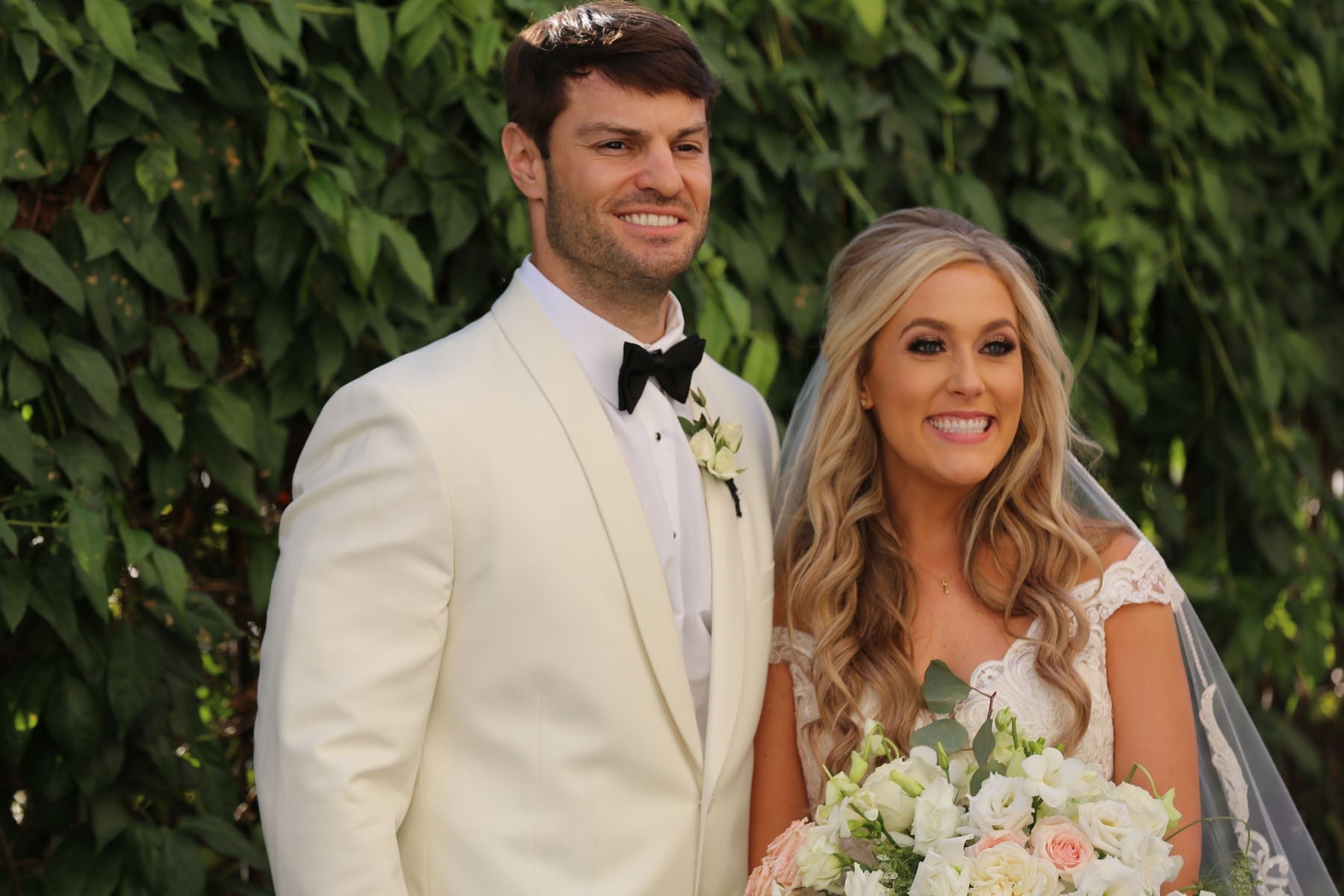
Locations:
[918,561,961,596]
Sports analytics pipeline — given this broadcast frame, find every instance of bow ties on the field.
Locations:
[618,334,706,415]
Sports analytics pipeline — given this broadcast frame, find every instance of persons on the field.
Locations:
[748,208,1339,896]
[253,0,780,896]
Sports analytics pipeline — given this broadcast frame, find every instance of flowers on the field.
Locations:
[745,660,1263,896]
[676,386,749,517]
